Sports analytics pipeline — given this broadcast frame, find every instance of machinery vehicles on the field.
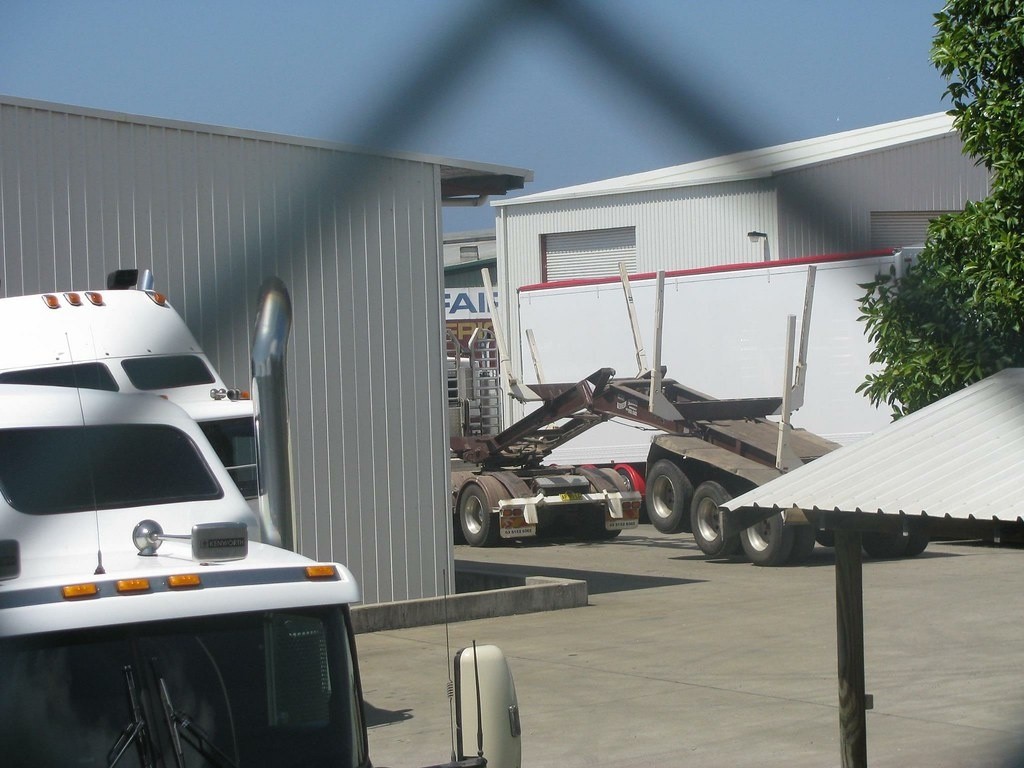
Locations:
[1,279,489,767]
[0,269,261,519]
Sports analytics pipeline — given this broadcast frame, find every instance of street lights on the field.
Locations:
[747,231,771,261]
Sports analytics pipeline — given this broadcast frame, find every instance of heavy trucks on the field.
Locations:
[445,262,929,567]
